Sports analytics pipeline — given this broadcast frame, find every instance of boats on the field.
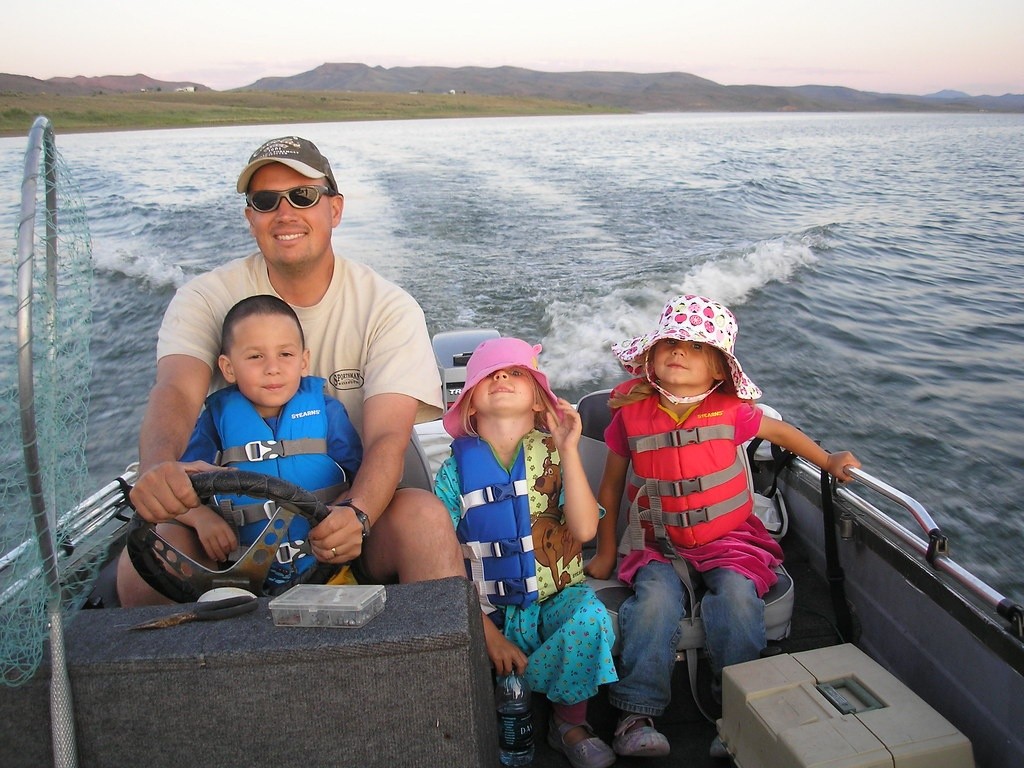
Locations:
[0,328,1024,768]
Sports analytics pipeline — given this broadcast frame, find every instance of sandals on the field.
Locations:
[710,732,729,755]
[613,713,669,753]
[547,715,615,768]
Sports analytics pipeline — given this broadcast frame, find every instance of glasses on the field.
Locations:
[245,186,338,214]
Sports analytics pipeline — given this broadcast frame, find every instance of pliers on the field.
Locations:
[126,594,259,637]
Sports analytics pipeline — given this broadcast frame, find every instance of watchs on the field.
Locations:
[335,497,370,542]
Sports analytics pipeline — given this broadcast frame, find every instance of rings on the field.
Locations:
[331,547,337,558]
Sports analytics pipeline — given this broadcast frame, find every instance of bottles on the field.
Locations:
[497,665,534,768]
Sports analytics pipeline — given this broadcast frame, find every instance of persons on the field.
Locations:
[584,294,862,756]
[116,135,467,605]
[432,338,619,768]
[175,295,362,597]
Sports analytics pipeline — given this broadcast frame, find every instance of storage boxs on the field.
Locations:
[269,584,387,629]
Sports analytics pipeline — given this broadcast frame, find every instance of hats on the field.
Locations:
[611,291,763,402]
[442,337,564,437]
[237,138,338,194]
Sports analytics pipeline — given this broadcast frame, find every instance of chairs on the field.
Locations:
[576,389,794,660]
